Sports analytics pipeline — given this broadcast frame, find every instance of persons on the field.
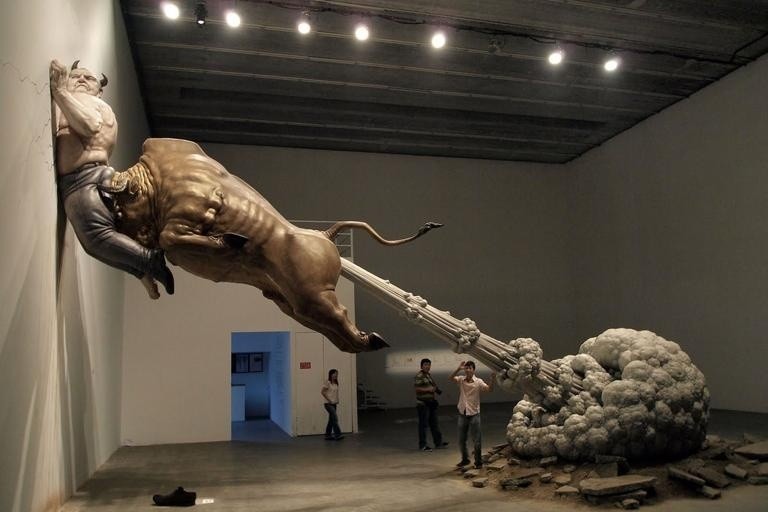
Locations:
[320,368,346,441]
[49,58,175,300]
[448,359,497,470]
[412,358,451,453]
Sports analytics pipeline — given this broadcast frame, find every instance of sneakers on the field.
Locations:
[435,442,449,448]
[474,464,483,469]
[457,459,470,466]
[421,446,433,452]
[153,486,196,506]
[324,435,345,440]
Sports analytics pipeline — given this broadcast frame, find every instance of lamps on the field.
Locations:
[192,0,208,26]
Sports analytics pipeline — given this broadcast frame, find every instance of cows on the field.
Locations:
[96,134,445,354]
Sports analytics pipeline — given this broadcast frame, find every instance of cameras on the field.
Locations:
[434,387,442,395]
